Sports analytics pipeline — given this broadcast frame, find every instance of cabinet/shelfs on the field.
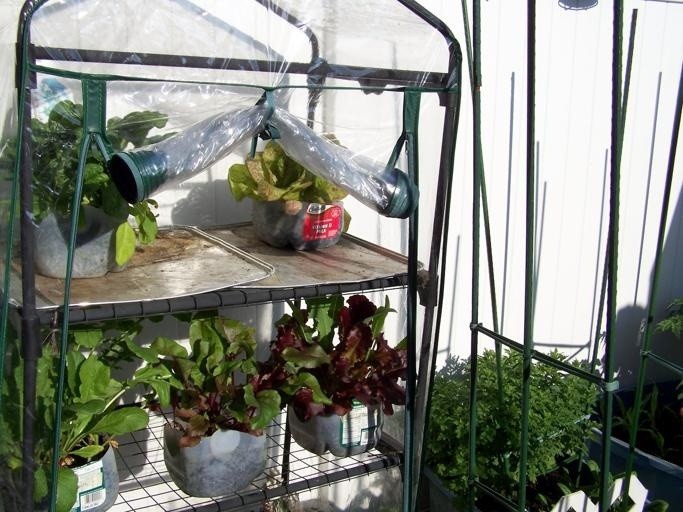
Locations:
[14,1,464,512]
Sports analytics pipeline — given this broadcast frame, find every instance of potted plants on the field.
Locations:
[227,133,359,252]
[3,289,407,511]
[2,101,177,280]
